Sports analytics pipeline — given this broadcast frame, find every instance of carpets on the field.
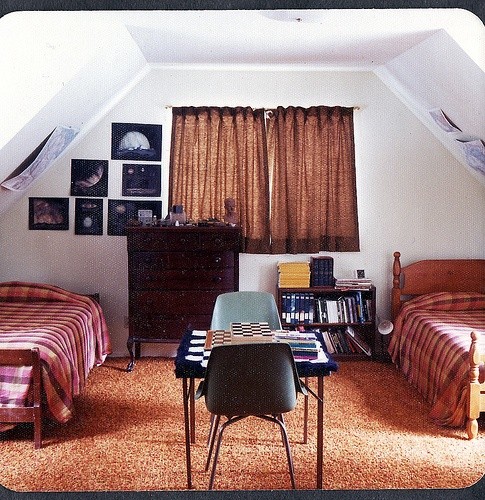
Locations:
[0,357,485,491]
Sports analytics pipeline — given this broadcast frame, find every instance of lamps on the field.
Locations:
[377,316,394,364]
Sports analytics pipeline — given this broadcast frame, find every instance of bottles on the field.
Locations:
[153,215,157,227]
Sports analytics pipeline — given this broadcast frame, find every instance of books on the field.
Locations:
[204,321,373,360]
[278,255,374,324]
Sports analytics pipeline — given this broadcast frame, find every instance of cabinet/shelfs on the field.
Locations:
[124,225,240,372]
[277,283,377,362]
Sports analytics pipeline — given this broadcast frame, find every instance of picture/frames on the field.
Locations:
[111,122,162,161]
[74,198,103,235]
[121,163,161,197]
[107,199,163,236]
[355,269,365,279]
[28,197,69,231]
[70,158,109,197]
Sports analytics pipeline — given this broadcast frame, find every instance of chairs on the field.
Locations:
[202,342,308,490]
[199,292,287,447]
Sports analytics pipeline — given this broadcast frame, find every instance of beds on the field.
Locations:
[0,282,116,449]
[387,252,485,440]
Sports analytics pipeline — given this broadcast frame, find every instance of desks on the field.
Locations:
[173,326,342,489]
